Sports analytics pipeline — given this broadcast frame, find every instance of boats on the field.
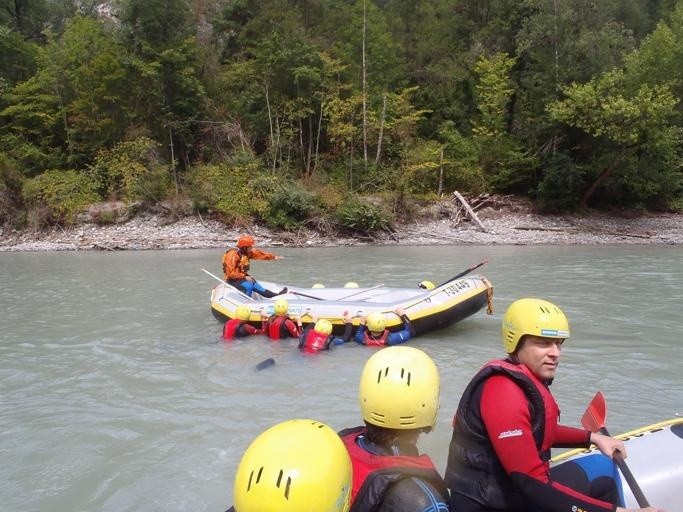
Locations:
[551,412,683,512]
[211,274,494,344]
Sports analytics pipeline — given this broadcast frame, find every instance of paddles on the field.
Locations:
[581,392,650,508]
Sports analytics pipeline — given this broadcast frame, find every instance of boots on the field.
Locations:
[263,287,287,298]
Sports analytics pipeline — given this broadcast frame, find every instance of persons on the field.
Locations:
[266,300,304,340]
[222,305,268,340]
[444,299,664,512]
[337,346,452,512]
[298,311,352,353]
[354,308,416,346]
[222,236,287,298]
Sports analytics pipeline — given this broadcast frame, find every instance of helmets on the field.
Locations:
[273,299,287,314]
[236,236,255,248]
[359,345,440,431]
[417,280,435,291]
[365,313,385,332]
[502,298,570,354]
[311,283,325,288]
[235,305,250,321]
[343,282,359,288]
[313,319,332,335]
